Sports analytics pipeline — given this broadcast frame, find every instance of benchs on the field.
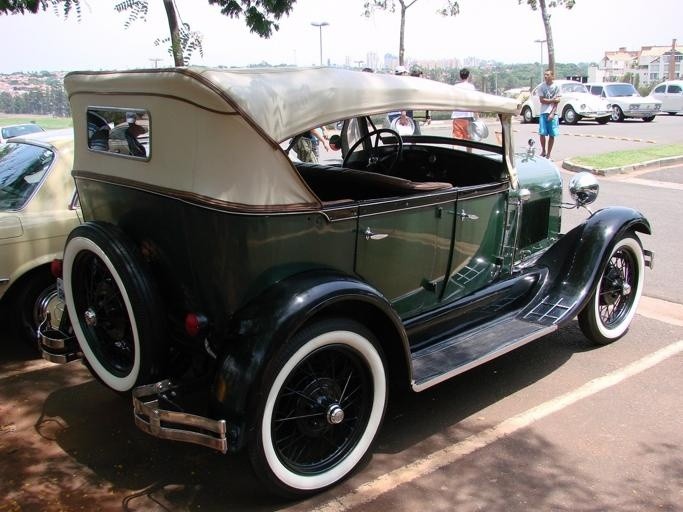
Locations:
[291,160,453,197]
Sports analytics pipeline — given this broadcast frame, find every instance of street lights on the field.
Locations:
[534,39,546,83]
[309,21,330,67]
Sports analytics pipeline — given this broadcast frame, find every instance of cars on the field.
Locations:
[0,123,84,350]
[520,80,682,124]
[36,68,656,501]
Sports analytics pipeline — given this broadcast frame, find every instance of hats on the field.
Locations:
[394,66,409,74]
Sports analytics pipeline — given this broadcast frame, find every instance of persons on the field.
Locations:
[312,126,329,162]
[342,66,431,150]
[449,69,479,153]
[536,69,562,162]
[290,128,330,164]
